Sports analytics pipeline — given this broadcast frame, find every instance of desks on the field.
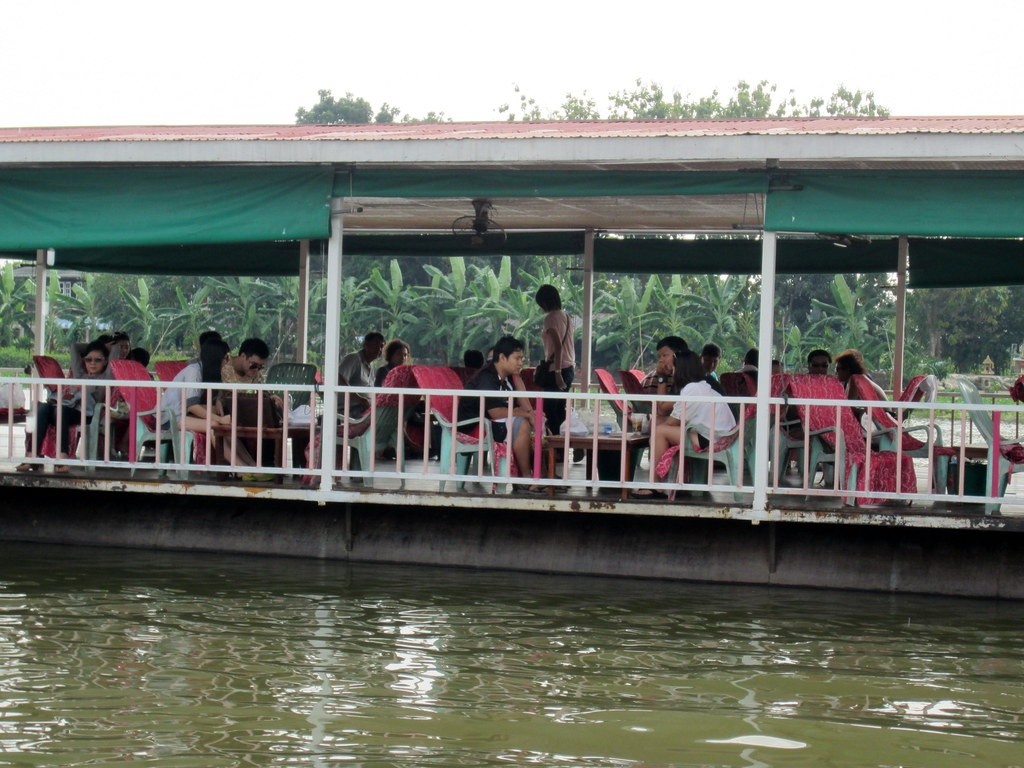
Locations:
[211,425,310,484]
[545,431,649,497]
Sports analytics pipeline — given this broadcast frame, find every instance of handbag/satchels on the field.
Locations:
[560,407,588,435]
[532,360,549,387]
[224,391,283,428]
[288,405,318,426]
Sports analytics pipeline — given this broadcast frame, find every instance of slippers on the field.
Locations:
[630,488,668,498]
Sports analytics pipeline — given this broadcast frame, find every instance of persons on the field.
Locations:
[631,335,758,500]
[772,349,891,473]
[536,284,576,436]
[96,331,155,458]
[455,337,571,494]
[161,332,283,482]
[337,332,410,460]
[15,341,121,472]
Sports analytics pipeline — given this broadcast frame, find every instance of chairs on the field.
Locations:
[0,341,1024,514]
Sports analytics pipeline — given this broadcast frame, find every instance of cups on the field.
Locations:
[600,414,614,433]
[631,413,644,434]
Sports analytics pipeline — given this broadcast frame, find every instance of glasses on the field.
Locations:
[84,357,106,363]
[486,357,494,364]
[672,351,680,359]
[247,359,265,371]
[810,362,829,369]
[837,366,849,372]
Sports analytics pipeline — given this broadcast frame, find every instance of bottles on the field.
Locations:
[288,392,292,411]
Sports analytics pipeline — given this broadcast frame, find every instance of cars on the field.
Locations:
[51,306,115,332]
[151,307,184,318]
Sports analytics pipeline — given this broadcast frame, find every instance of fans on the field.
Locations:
[452,199,506,249]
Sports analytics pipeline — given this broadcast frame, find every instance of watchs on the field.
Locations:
[555,369,561,373]
[657,377,668,384]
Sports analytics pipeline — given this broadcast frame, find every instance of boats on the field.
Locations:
[0,115,1024,602]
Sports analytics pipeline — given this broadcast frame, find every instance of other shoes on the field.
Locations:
[54,465,68,472]
[243,472,273,481]
[517,482,547,494]
[539,485,566,493]
[383,448,396,459]
[16,464,38,471]
[573,448,584,462]
[429,450,438,460]
